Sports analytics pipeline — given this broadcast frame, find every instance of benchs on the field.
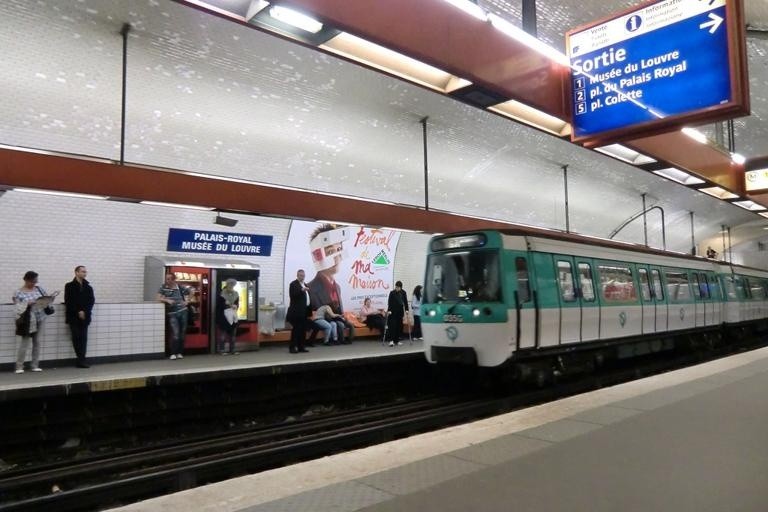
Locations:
[259,325,383,344]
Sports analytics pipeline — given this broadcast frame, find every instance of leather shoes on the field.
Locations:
[290,345,309,353]
[76,358,90,368]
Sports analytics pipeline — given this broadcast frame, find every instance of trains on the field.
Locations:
[413,227,768,386]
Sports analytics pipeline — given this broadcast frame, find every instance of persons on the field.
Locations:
[360,298,388,341]
[63,265,95,368]
[581,271,596,301]
[288,269,313,353]
[410,285,424,341]
[156,272,194,360]
[285,301,355,346]
[306,223,346,314]
[560,273,574,300]
[12,270,54,374]
[215,278,240,355]
[387,281,409,346]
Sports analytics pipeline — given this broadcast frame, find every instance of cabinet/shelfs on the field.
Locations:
[176,279,202,328]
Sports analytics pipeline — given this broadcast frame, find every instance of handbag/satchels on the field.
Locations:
[44,306,55,315]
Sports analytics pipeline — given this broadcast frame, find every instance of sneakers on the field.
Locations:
[389,340,403,346]
[170,353,183,360]
[15,370,24,373]
[32,368,42,372]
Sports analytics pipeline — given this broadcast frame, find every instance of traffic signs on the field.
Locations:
[564,0,744,145]
